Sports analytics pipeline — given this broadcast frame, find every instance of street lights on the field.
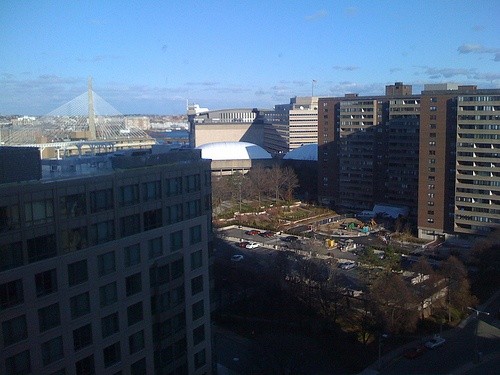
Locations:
[373,331,390,369]
[466,306,490,362]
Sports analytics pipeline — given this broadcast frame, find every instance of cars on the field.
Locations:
[403,345,427,358]
[247,230,275,237]
[231,254,243,262]
[283,236,299,242]
[377,253,385,259]
[426,336,447,350]
[340,262,355,270]
[240,242,261,249]
